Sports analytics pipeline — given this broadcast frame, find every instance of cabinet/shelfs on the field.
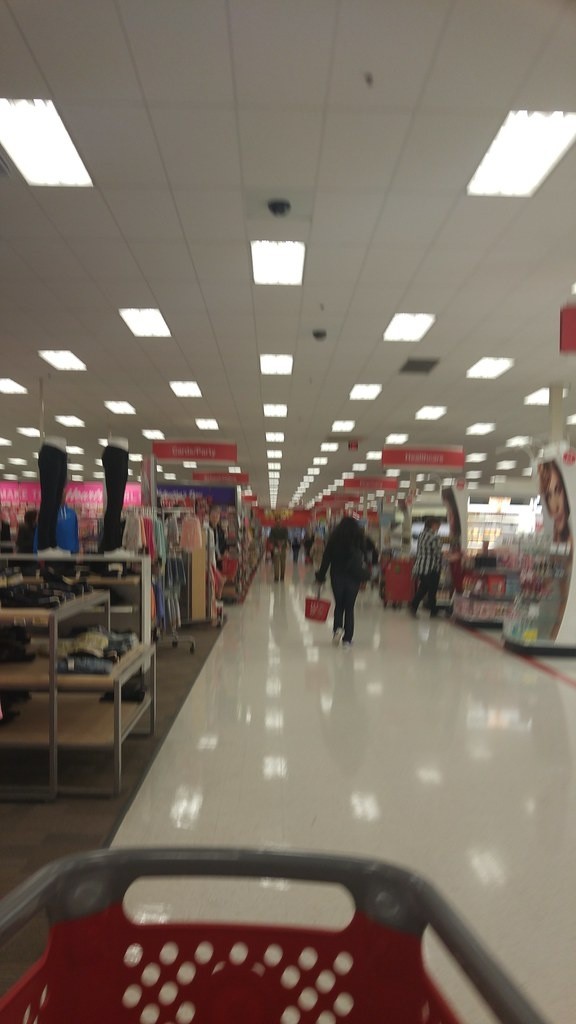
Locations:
[0,438,576,800]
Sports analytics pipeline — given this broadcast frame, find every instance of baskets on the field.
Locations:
[304,597,331,621]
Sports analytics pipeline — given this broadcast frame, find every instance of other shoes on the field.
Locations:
[343,641,353,647]
[332,628,343,647]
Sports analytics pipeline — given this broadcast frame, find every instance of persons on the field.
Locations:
[101,437,131,556]
[37,435,70,556]
[0,520,13,573]
[315,517,378,647]
[33,490,79,581]
[292,536,300,562]
[303,534,313,560]
[268,514,288,582]
[210,510,225,572]
[442,487,461,552]
[538,462,571,541]
[16,509,38,580]
[409,516,443,617]
